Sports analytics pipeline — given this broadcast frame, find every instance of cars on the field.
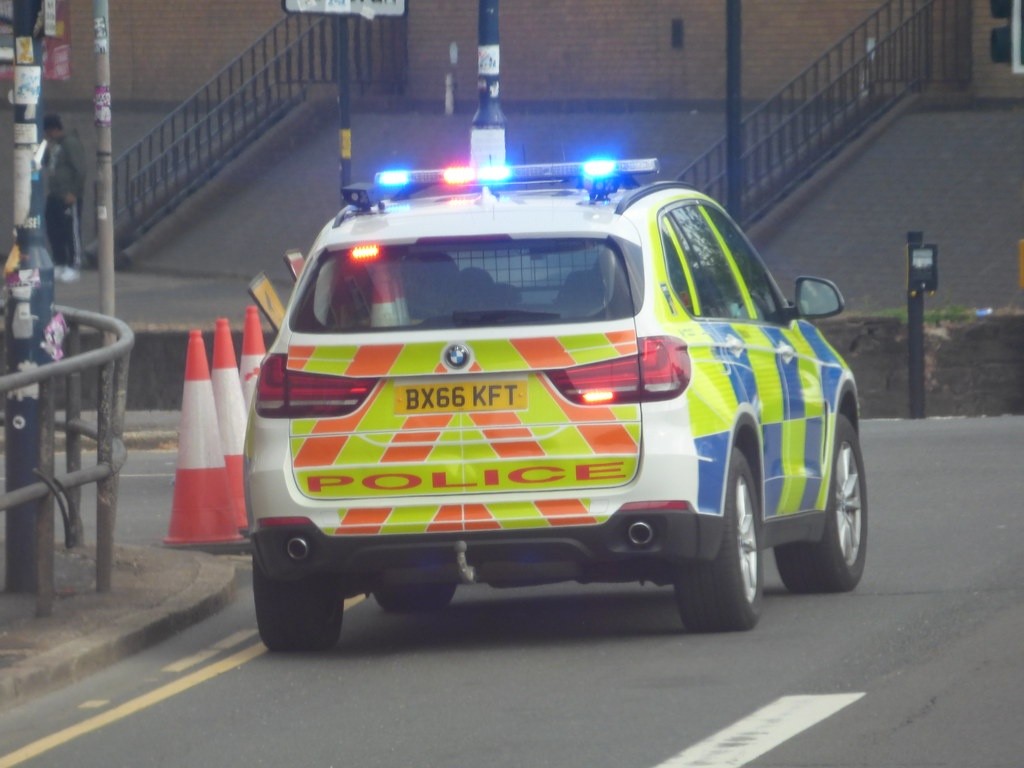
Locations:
[240,159,870,655]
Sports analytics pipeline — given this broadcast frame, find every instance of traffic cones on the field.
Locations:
[239,304,266,408]
[158,328,250,551]
[210,316,251,535]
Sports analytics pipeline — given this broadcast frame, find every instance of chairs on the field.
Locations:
[456,267,505,307]
[555,273,603,306]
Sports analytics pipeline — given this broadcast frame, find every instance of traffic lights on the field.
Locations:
[990,0,1024,75]
